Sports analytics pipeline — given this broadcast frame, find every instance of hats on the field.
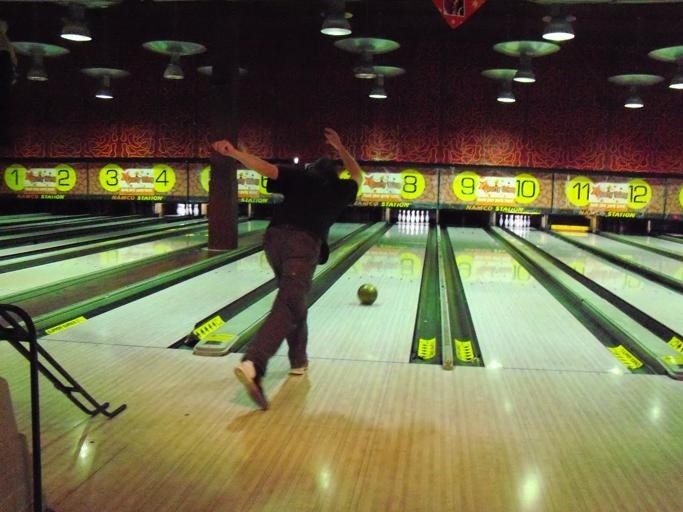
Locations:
[315,157,344,168]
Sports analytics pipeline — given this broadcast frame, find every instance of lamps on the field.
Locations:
[316,2,413,101]
[606,31,682,109]
[474,3,577,104]
[12,4,222,102]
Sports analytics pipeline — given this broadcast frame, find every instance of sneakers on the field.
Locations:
[288,358,309,375]
[234,359,270,410]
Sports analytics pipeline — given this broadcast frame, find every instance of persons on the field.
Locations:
[210,127,363,412]
